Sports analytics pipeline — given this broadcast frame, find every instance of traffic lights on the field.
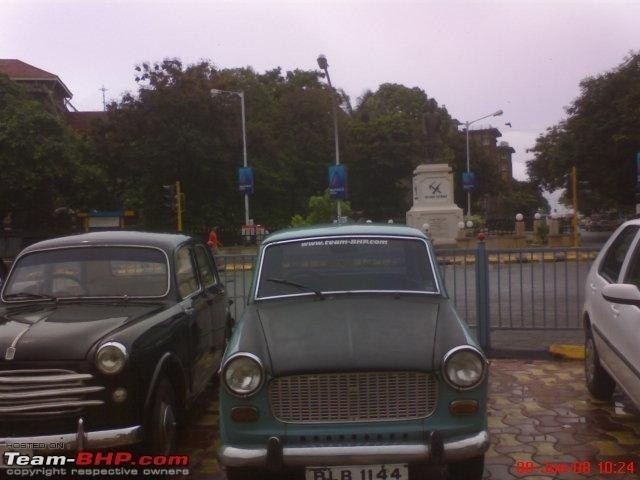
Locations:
[158,183,177,215]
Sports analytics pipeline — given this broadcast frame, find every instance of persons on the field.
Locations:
[208,227,223,254]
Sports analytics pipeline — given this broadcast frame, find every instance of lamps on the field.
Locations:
[421,210,559,234]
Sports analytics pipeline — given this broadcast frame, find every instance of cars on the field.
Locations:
[0,229,233,480]
[579,217,640,412]
[214,223,490,480]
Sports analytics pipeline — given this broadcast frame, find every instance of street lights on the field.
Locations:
[209,86,252,248]
[466,109,505,220]
[315,53,343,223]
